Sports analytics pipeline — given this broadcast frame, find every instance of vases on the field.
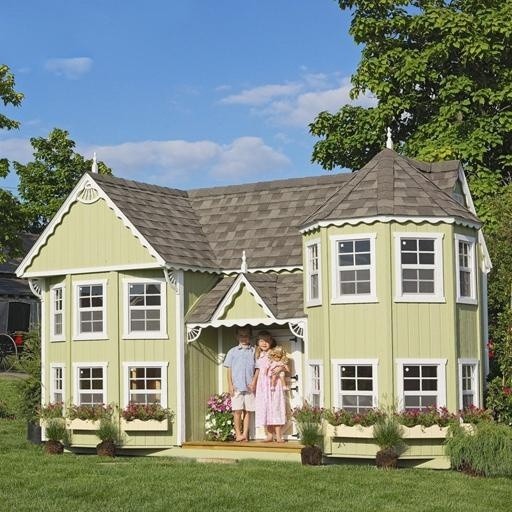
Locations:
[205,392,236,441]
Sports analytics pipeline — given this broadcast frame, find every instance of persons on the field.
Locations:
[222,325,255,441]
[247,330,286,443]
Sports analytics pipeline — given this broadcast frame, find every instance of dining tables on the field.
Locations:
[294,414,327,466]
[372,391,408,470]
[27,406,42,444]
[95,413,121,456]
[45,419,66,453]
